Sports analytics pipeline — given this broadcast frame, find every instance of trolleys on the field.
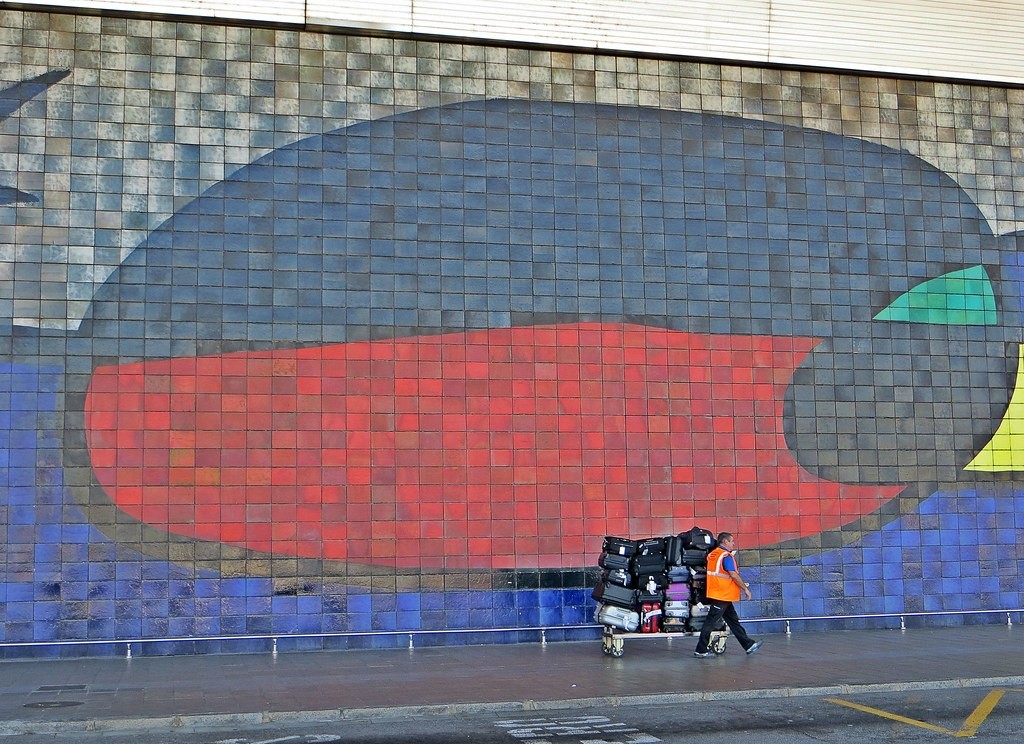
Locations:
[601,550,736,658]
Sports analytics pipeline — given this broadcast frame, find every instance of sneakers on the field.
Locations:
[694,652,710,658]
[746,639,764,656]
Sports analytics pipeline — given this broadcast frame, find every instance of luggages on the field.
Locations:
[591,526,726,633]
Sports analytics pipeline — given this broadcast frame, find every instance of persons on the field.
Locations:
[693,532,764,658]
